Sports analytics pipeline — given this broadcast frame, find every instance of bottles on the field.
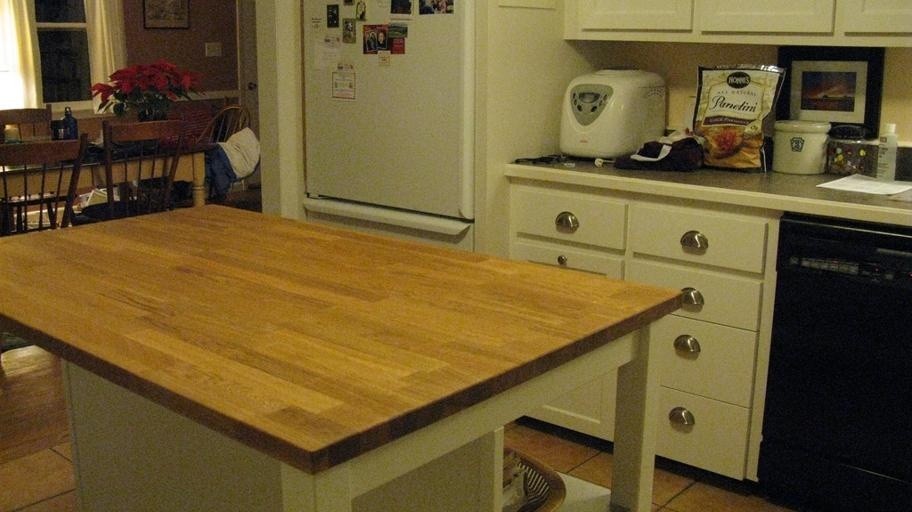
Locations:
[827,121,870,177]
[61,106,79,140]
[877,122,897,182]
[771,119,831,176]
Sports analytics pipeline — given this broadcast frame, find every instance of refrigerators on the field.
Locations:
[302,0,476,257]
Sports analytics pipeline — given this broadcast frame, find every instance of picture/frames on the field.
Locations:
[776,45,885,139]
[142,0,191,30]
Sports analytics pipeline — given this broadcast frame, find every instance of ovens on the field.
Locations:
[756,211,911,512]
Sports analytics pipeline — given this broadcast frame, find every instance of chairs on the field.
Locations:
[1,103,251,236]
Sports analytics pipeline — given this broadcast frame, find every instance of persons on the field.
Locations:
[377,31,386,48]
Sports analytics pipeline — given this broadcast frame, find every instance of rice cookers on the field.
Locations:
[558,63,669,165]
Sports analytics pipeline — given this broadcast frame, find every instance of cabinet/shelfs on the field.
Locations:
[504,179,784,485]
[562,0,912,47]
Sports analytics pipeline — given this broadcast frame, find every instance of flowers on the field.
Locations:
[85,58,206,118]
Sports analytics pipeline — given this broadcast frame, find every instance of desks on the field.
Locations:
[1,204,687,512]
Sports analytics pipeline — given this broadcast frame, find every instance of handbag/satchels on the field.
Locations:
[613,129,705,172]
